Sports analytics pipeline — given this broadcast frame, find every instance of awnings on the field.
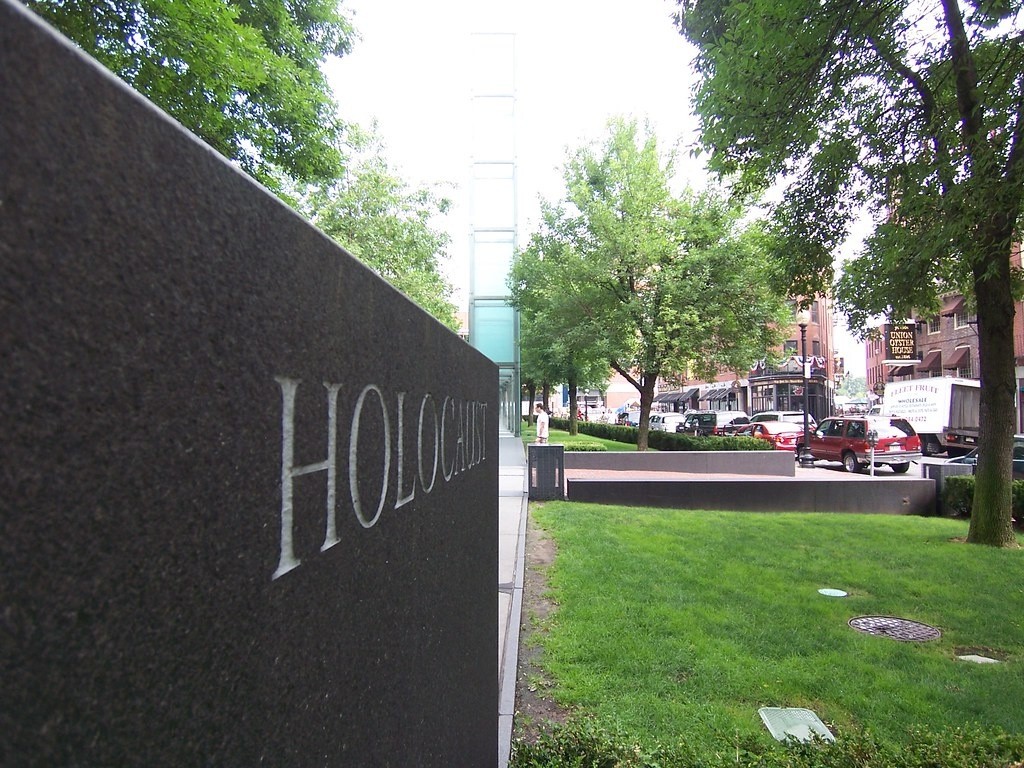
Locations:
[652,388,733,403]
[889,296,969,377]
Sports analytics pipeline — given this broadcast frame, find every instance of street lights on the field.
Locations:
[796,308,816,470]
[584,388,589,422]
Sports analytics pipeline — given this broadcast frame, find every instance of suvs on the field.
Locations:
[797,414,923,473]
[748,409,818,434]
[675,410,749,437]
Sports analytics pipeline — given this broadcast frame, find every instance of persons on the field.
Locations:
[535,403,549,444]
[577,409,612,424]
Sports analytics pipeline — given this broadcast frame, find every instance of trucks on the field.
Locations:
[867,377,982,459]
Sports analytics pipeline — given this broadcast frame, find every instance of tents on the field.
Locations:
[845,397,871,413]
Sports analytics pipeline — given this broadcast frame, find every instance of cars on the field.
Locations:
[943,435,1024,480]
[618,412,639,427]
[648,412,685,433]
[717,415,752,435]
[734,421,805,452]
